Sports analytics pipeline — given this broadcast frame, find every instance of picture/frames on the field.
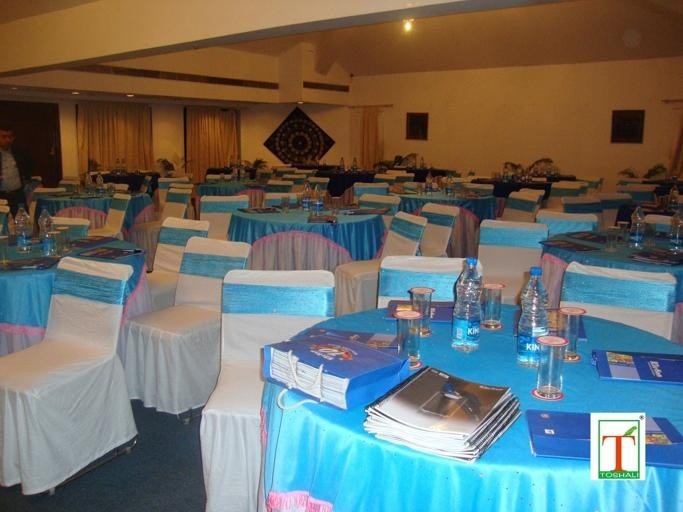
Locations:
[405,112,429,141]
[611,109,644,144]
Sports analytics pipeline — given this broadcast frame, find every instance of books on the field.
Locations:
[539,231,681,266]
[364,367,522,464]
[384,300,459,323]
[263,335,410,411]
[2,255,62,271]
[78,247,145,261]
[236,203,390,222]
[525,409,683,468]
[594,348,682,384]
[291,328,399,357]
[72,234,119,249]
[513,310,587,339]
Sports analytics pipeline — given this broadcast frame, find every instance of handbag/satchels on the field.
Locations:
[262,328,410,410]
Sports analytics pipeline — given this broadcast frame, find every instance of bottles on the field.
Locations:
[452,256,483,353]
[627,202,646,249]
[503,164,523,182]
[516,267,549,368]
[445,172,453,198]
[669,202,682,253]
[85,171,104,197]
[351,156,358,176]
[39,204,55,257]
[301,179,325,217]
[425,171,433,196]
[339,157,346,176]
[14,203,35,253]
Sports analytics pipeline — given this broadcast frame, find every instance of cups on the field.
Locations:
[557,312,581,361]
[604,220,681,254]
[0,220,72,264]
[395,318,421,367]
[482,289,502,330]
[538,342,567,398]
[409,291,432,335]
